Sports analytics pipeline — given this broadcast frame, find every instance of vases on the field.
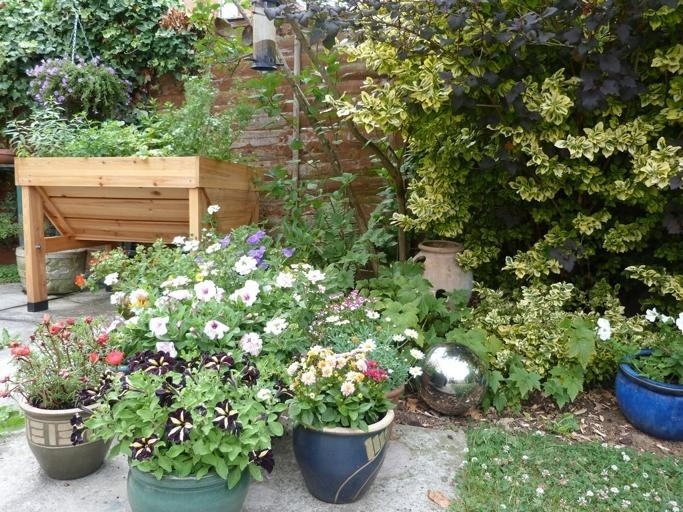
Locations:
[408,238,476,311]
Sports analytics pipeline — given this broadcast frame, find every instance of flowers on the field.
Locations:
[595,305,682,385]
[23,53,135,120]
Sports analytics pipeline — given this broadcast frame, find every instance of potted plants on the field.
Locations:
[614,348,681,443]
